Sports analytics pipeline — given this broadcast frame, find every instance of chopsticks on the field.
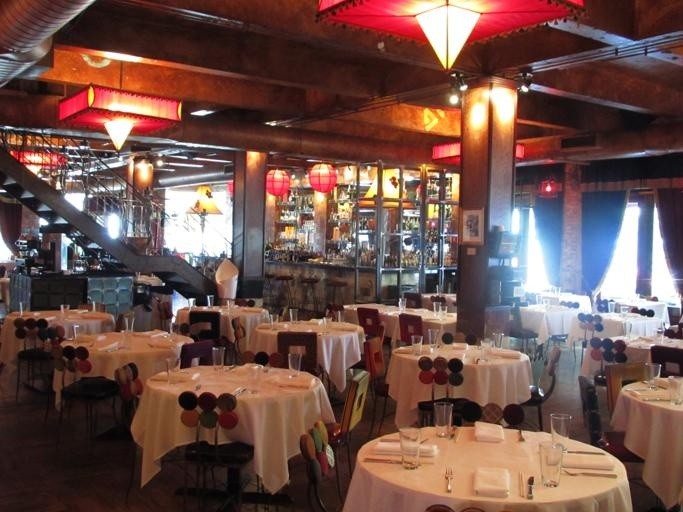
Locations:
[363,456,427,465]
[454,427,462,443]
[519,472,525,497]
[380,437,430,445]
[565,451,605,455]
[563,469,618,479]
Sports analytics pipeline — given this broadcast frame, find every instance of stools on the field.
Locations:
[299,278,320,312]
[264,272,274,306]
[276,275,294,307]
[325,277,348,305]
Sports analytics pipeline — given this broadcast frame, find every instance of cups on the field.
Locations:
[433,302,441,316]
[633,294,640,304]
[323,317,331,330]
[621,306,629,317]
[339,310,346,324]
[188,297,196,310]
[72,325,80,337]
[495,333,504,350]
[554,287,561,295]
[288,353,301,377]
[19,301,29,317]
[542,299,550,310]
[481,341,489,359]
[433,402,454,437]
[271,314,279,330]
[398,297,406,312]
[207,295,214,308]
[535,295,542,306]
[669,375,682,405]
[125,317,134,332]
[429,329,439,348]
[645,363,661,391]
[166,358,181,384]
[654,328,663,344]
[399,426,420,469]
[290,309,297,323]
[440,306,448,319]
[609,302,616,313]
[228,301,234,310]
[551,413,569,452]
[212,346,225,370]
[412,335,422,352]
[61,304,70,317]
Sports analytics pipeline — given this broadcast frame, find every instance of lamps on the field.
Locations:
[186,186,224,232]
[315,0,586,74]
[432,140,529,169]
[11,151,68,176]
[58,60,182,153]
[360,168,416,209]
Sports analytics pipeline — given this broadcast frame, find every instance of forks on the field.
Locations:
[519,428,524,442]
[539,442,561,487]
[561,463,613,471]
[445,467,455,492]
[527,476,534,500]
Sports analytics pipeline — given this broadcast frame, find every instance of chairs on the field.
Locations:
[235,299,256,306]
[36,325,66,427]
[13,317,49,407]
[171,322,202,340]
[49,344,119,449]
[579,312,603,365]
[78,303,106,313]
[430,295,445,304]
[115,362,145,506]
[664,328,683,340]
[579,375,645,463]
[240,350,283,368]
[178,390,253,511]
[357,306,379,336]
[353,320,386,369]
[301,422,344,511]
[399,312,423,345]
[631,307,655,317]
[507,305,540,353]
[606,362,651,413]
[640,294,660,300]
[115,310,134,331]
[324,303,345,322]
[277,331,317,375]
[651,344,683,377]
[403,291,421,308]
[590,337,638,386]
[325,370,370,505]
[560,300,579,310]
[461,401,523,427]
[180,341,215,369]
[189,310,220,341]
[363,336,390,438]
[596,299,615,312]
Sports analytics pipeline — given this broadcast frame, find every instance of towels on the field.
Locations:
[90,332,107,341]
[399,345,433,353]
[474,419,505,443]
[212,384,241,395]
[632,389,670,399]
[13,310,37,316]
[94,341,120,350]
[562,453,614,469]
[447,311,457,317]
[448,351,465,363]
[424,315,439,321]
[452,342,468,349]
[335,323,358,331]
[149,339,176,349]
[494,350,522,359]
[70,306,89,313]
[474,466,511,497]
[150,369,201,382]
[374,441,438,457]
[149,330,167,338]
[308,317,323,326]
[216,258,238,308]
[280,377,316,389]
[655,374,679,387]
[257,322,271,329]
[42,314,57,321]
[243,305,265,314]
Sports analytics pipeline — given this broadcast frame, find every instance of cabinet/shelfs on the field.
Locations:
[326,185,385,258]
[400,200,454,256]
[271,185,314,252]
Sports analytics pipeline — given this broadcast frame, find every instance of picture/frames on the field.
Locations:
[458,206,486,247]
